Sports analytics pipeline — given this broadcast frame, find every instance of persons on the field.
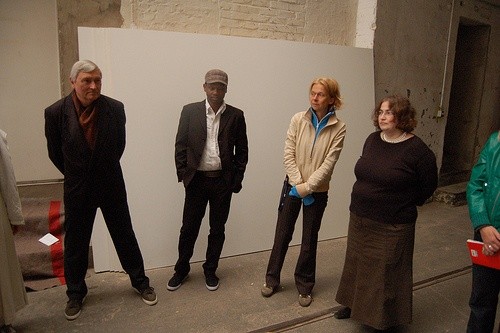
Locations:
[334,94,438,333]
[466,131,500,333]
[0,130,28,333]
[43,60,158,319]
[166,69,249,290]
[262,76,347,307]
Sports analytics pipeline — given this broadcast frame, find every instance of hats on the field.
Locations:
[204,69,228,86]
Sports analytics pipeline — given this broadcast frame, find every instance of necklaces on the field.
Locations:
[384,132,406,143]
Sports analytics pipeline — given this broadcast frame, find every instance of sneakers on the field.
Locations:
[64,289,88,320]
[261,283,281,298]
[134,286,158,306]
[298,292,313,307]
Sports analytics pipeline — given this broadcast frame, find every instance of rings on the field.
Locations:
[488,245,491,248]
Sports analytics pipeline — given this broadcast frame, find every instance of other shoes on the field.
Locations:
[203,269,220,291]
[167,271,189,290]
[334,307,352,319]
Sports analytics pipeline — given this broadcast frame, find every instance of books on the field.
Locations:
[466,238,500,270]
